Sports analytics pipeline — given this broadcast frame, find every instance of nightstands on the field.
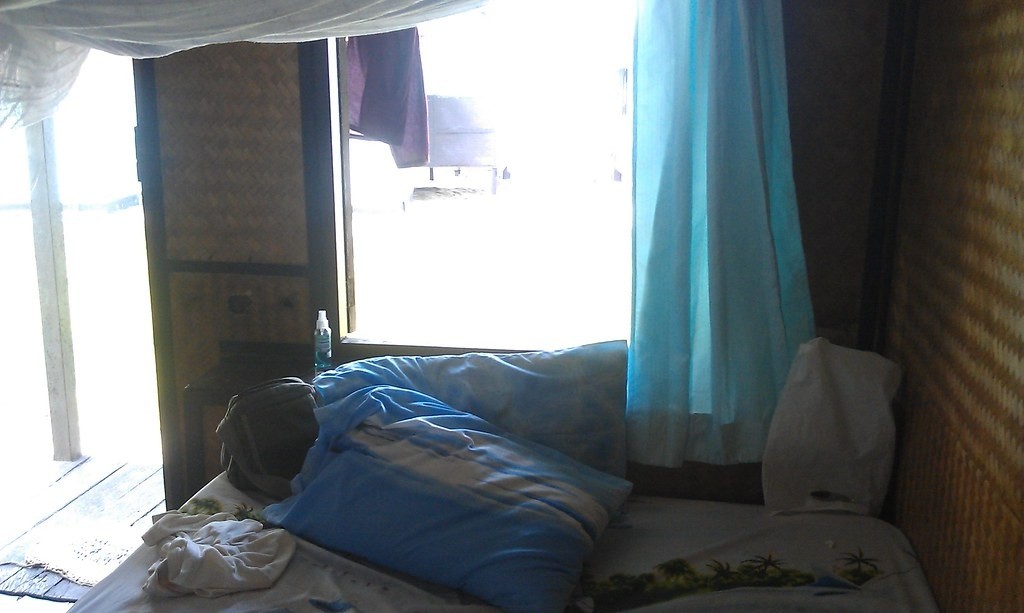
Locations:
[183,359,305,497]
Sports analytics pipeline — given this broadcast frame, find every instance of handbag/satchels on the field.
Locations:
[762,336,903,519]
[214,376,319,498]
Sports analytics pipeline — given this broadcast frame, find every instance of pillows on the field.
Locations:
[255,386,632,613]
[314,340,628,481]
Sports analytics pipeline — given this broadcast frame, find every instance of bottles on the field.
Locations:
[313,311,332,367]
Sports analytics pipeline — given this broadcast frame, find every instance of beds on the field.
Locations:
[69,344,939,613]
